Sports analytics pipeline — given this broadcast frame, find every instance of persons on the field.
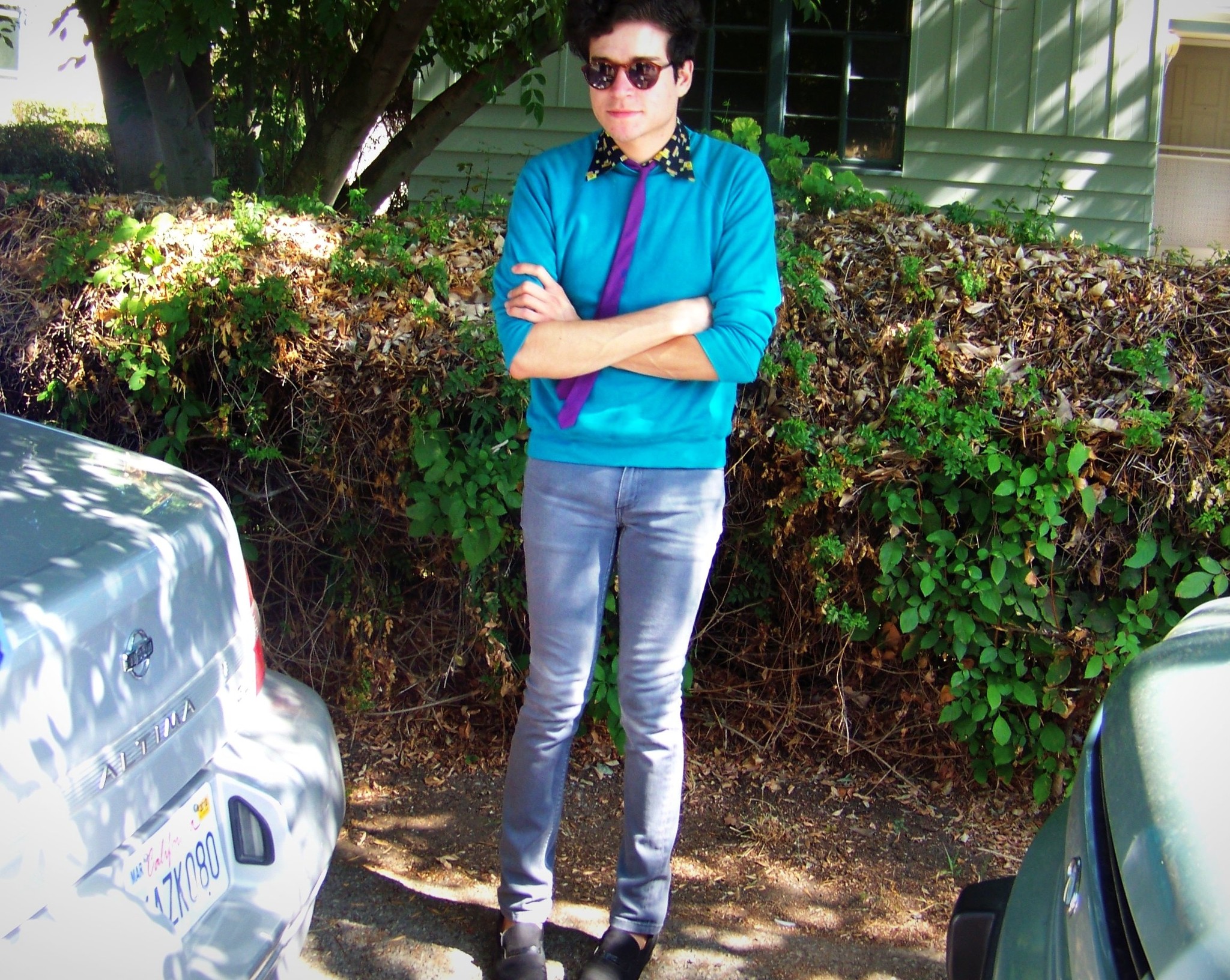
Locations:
[493,0,780,980]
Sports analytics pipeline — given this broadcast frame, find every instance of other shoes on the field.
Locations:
[580,927,658,979]
[496,913,547,980]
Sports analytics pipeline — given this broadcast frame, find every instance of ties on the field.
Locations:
[558,159,661,428]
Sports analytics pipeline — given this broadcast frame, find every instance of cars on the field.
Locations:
[1,404,348,980]
[937,592,1229,980]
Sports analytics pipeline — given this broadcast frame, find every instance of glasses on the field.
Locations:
[581,59,674,90]
